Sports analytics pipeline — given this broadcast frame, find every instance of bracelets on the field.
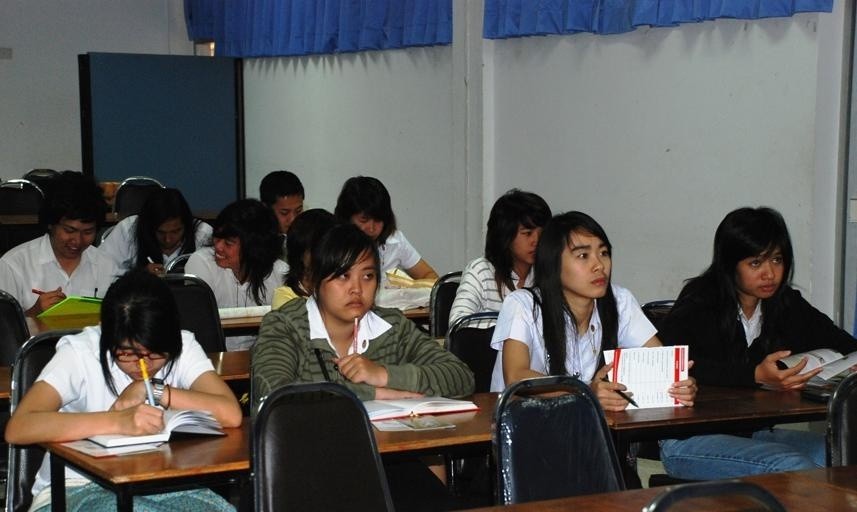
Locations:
[164,382,174,412]
[144,377,164,407]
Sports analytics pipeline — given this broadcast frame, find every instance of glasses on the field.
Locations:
[107,345,172,362]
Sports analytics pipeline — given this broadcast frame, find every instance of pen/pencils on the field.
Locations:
[216,351,224,375]
[354,318,358,354]
[138,358,160,433]
[32,288,45,295]
[599,377,639,408]
[147,256,158,271]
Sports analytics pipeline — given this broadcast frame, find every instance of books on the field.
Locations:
[86,407,227,449]
[359,395,481,421]
[776,347,856,389]
[147,248,200,275]
[33,294,103,323]
[600,345,689,411]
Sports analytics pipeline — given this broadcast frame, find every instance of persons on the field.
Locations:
[257,169,305,265]
[332,176,442,290]
[1,266,244,511]
[486,207,697,414]
[245,216,483,511]
[654,204,857,486]
[98,186,216,282]
[0,170,121,318]
[182,196,290,354]
[271,205,336,313]
[447,187,554,333]
